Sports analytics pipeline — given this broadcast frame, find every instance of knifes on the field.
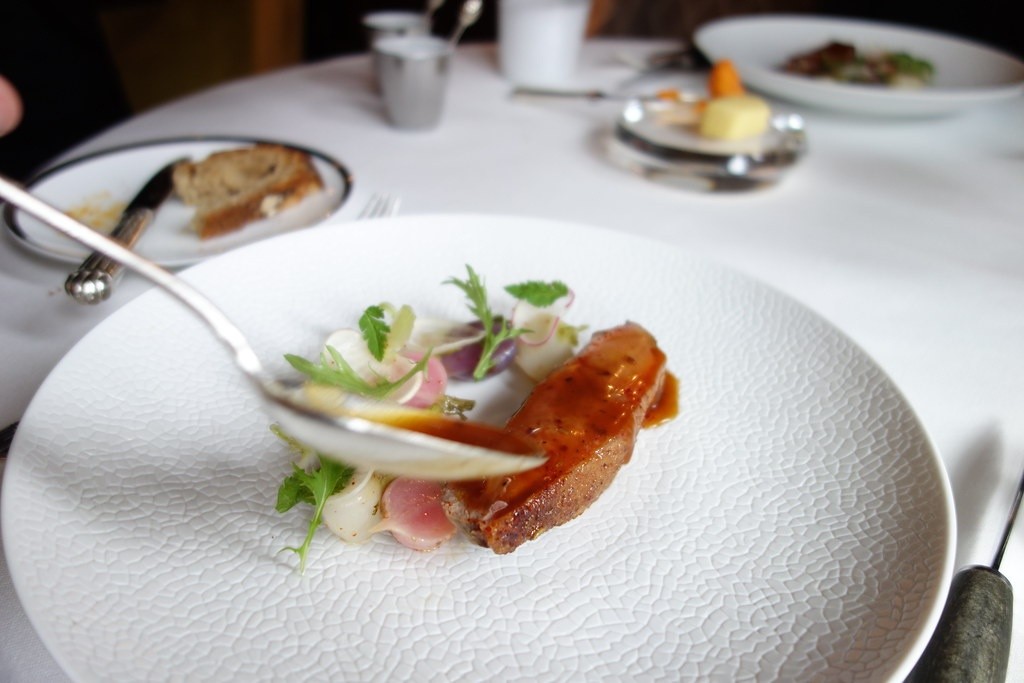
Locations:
[64,155,190,303]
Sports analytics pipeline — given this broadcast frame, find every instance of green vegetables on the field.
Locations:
[271,264,575,570]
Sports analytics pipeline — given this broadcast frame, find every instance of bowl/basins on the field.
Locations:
[613,67,805,193]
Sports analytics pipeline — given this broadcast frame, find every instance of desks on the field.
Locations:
[0,37,1024,683]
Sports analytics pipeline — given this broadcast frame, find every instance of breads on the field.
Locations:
[171,144,326,240]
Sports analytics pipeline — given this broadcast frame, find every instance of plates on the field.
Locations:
[693,14,1024,117]
[1,215,957,683]
[4,135,352,267]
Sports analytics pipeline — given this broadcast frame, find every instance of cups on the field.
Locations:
[363,12,432,95]
[498,0,591,86]
[373,37,450,130]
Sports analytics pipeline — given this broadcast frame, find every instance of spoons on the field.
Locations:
[0,176,548,479]
